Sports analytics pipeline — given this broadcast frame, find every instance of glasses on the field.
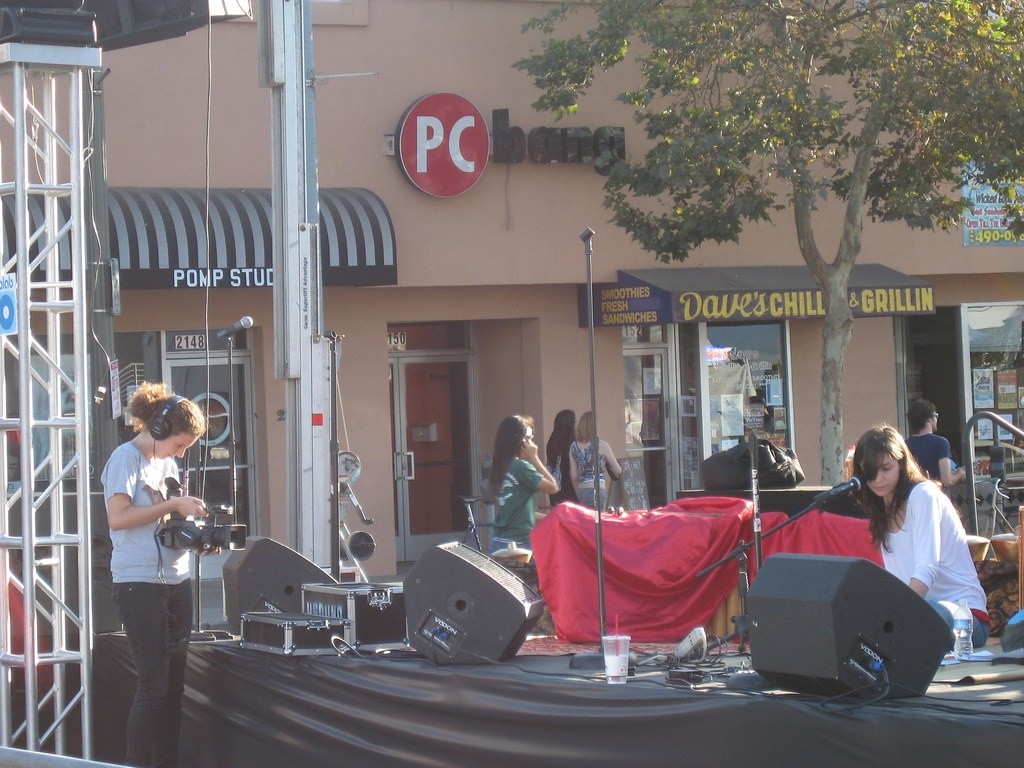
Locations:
[523,434,535,441]
[933,413,938,419]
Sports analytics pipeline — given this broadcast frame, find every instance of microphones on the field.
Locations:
[217,316,253,339]
[814,475,864,506]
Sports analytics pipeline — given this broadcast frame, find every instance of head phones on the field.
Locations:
[150,395,188,440]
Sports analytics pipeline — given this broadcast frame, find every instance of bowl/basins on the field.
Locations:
[990,539,1019,563]
[967,540,990,563]
[492,542,532,564]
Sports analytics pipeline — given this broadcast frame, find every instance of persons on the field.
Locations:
[484,415,559,573]
[851,427,990,650]
[546,410,622,513]
[101,381,210,768]
[903,400,966,493]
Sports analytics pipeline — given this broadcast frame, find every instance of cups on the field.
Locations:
[602,635,631,685]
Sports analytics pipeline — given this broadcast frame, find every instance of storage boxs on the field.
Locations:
[300,580,410,651]
[239,610,351,657]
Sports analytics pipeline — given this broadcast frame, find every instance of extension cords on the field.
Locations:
[674,627,705,659]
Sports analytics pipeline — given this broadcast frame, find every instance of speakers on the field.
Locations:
[223,537,338,635]
[403,540,545,666]
[745,553,956,701]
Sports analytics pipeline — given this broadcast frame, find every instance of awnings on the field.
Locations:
[577,264,935,329]
[0,187,399,287]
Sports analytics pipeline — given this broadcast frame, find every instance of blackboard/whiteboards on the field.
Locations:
[605,456,651,511]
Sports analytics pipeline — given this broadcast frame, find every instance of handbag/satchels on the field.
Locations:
[546,464,562,492]
[700,441,804,489]
[606,464,619,481]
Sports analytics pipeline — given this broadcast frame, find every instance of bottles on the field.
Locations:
[953,598,974,657]
